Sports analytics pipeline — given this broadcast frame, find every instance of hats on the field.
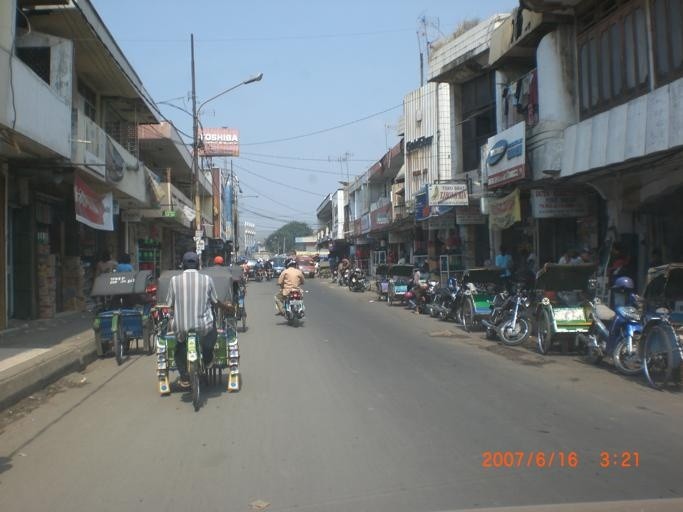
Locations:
[182,252,200,263]
[214,255,224,264]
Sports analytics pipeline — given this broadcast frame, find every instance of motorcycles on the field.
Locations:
[575,276,652,377]
[405,277,461,320]
[332,268,371,292]
[243,268,274,284]
[480,279,531,346]
[275,283,305,326]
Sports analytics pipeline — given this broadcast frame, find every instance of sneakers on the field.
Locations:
[204,352,215,369]
[180,379,191,388]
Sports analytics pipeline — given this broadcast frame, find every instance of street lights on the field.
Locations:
[190,31,264,271]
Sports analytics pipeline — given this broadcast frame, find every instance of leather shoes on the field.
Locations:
[274,312,285,316]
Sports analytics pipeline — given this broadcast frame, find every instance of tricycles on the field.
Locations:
[231,264,248,331]
[636,261,682,389]
[386,264,415,305]
[529,260,599,354]
[375,263,389,301]
[90,268,158,364]
[458,266,509,332]
[149,266,240,411]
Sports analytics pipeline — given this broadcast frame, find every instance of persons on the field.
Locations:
[264,261,274,278]
[349,261,359,269]
[386,251,393,263]
[253,260,264,276]
[397,249,407,265]
[495,243,514,293]
[94,249,116,275]
[271,258,304,316]
[164,251,235,391]
[111,253,136,272]
[242,259,250,282]
[647,247,663,269]
[335,260,350,286]
[571,247,593,266]
[557,250,574,266]
[211,256,227,272]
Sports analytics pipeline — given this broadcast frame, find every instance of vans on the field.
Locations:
[246,255,316,278]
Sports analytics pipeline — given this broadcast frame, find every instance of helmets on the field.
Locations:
[404,291,415,299]
